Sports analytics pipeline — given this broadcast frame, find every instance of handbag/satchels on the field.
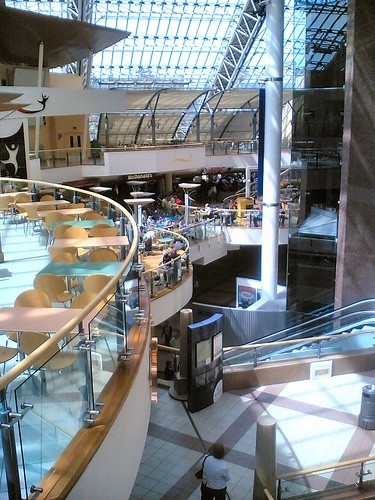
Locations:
[195,468,204,479]
[169,336,176,347]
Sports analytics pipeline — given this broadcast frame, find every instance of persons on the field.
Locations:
[164,361,173,380]
[161,325,172,345]
[139,173,258,289]
[201,444,230,500]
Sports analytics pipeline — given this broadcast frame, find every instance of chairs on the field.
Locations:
[0,187,121,397]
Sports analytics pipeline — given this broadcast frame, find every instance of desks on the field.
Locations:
[245,213,257,227]
[14,200,70,209]
[35,262,132,302]
[37,207,93,222]
[195,210,233,226]
[151,238,187,287]
[0,306,109,369]
[54,235,129,248]
[0,191,29,197]
[51,219,115,229]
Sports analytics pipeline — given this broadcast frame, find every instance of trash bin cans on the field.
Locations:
[357,384,375,429]
